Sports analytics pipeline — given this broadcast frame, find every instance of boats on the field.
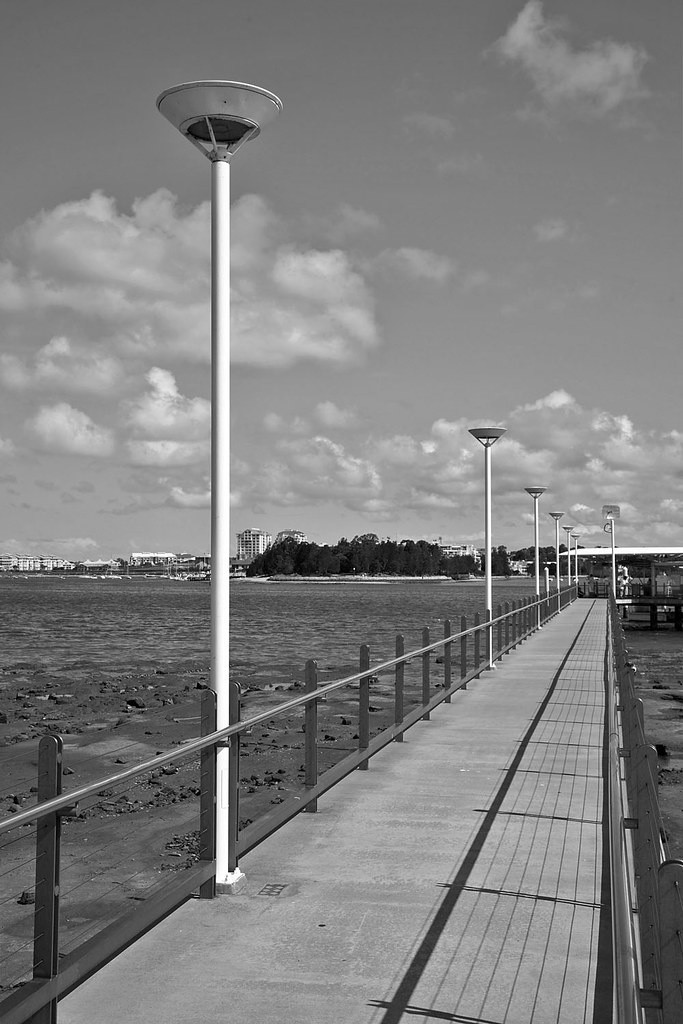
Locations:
[91,575,97,579]
[549,577,554,581]
[572,577,579,582]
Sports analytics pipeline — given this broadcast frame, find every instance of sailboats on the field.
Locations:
[101,556,207,582]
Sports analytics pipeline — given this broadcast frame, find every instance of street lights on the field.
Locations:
[466,426,508,668]
[549,512,564,614]
[571,533,581,599]
[526,485,547,630]
[562,526,574,605]
[603,505,620,599]
[155,79,282,891]
